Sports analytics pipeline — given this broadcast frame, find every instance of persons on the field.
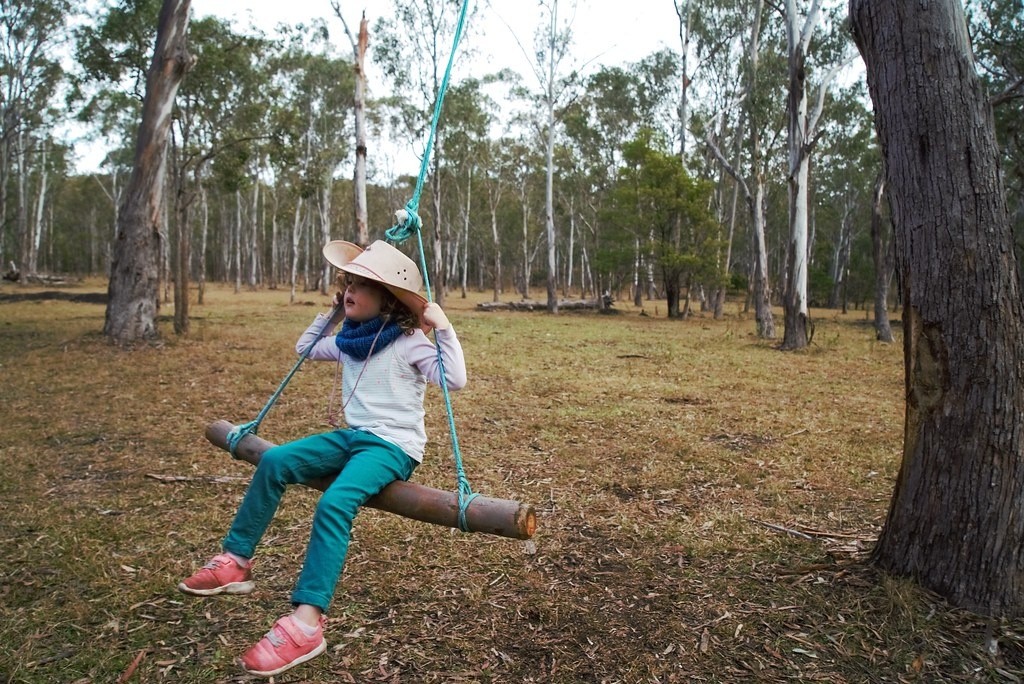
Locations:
[177,239,468,675]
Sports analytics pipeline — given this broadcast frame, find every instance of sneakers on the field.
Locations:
[179,554,257,595]
[238,616,328,677]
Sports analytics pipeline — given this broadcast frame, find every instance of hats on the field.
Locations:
[323,240,433,336]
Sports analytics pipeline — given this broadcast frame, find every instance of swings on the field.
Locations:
[203,1,536,540]
